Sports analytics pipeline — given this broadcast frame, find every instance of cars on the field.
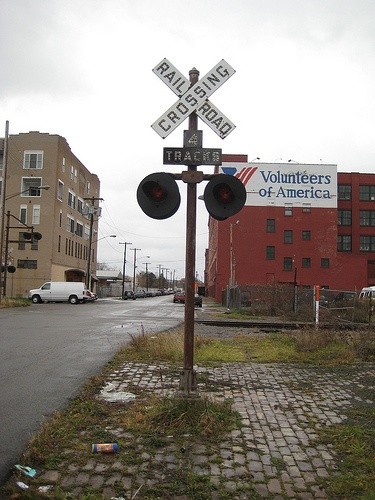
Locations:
[133,287,202,307]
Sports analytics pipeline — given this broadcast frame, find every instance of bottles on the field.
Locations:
[92,443,118,453]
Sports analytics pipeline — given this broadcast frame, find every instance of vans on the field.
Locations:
[358,285,375,302]
[28,281,99,304]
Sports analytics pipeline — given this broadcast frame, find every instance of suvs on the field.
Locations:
[124,290,136,300]
[333,290,357,303]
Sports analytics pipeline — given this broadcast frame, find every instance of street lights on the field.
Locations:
[87,234,117,290]
[133,256,151,291]
[0,184,51,305]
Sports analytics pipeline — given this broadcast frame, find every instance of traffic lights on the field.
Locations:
[137,172,181,219]
[203,174,247,218]
